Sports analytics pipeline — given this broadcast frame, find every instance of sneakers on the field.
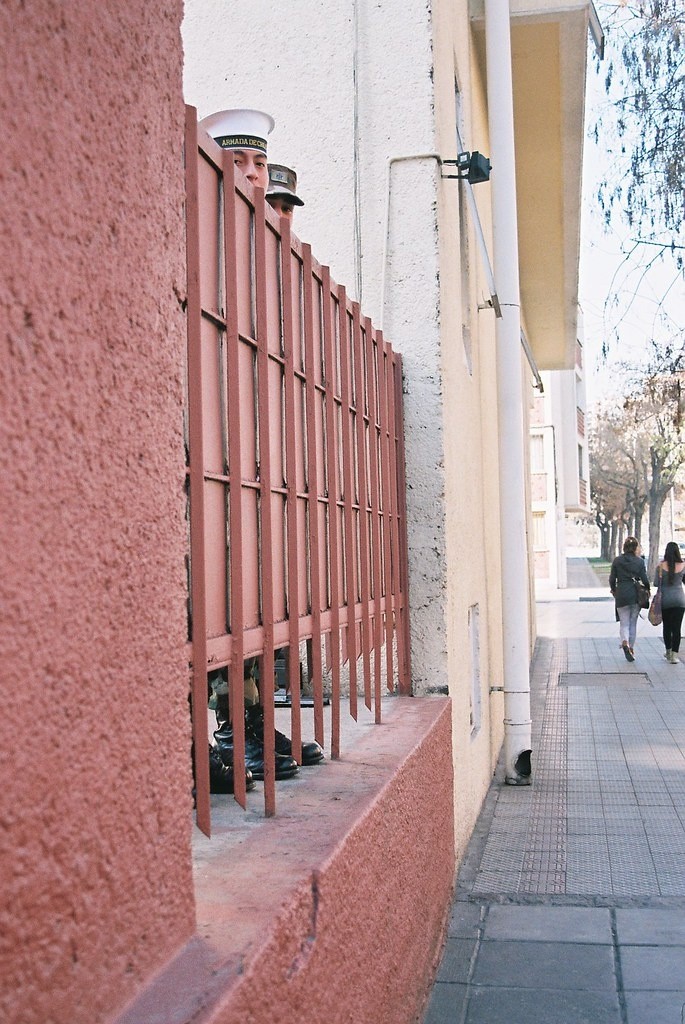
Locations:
[663,648,672,661]
[670,651,679,664]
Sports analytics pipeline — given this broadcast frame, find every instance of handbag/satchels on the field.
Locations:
[648,587,663,626]
[637,585,650,609]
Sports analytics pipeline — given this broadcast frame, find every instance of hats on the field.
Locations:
[263,163,305,209]
[197,106,277,158]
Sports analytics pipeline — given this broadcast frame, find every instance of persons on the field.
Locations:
[652,542,685,663]
[192,740,259,795]
[207,653,325,778]
[610,536,652,663]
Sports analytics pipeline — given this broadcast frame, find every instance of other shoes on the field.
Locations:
[622,640,635,663]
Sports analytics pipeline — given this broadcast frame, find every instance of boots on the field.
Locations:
[238,704,325,770]
[212,708,299,782]
[191,739,254,797]
